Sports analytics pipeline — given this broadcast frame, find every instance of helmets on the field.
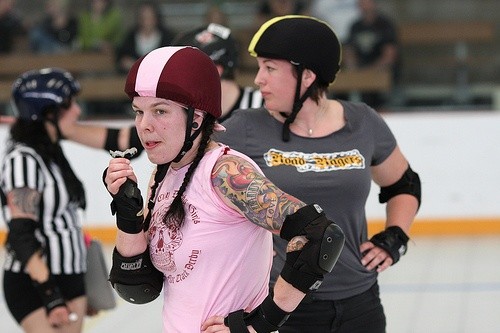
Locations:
[248,14,342,87]
[12,67,80,118]
[177,23,238,69]
[124,45,222,119]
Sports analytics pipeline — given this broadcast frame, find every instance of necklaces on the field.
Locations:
[286,100,330,136]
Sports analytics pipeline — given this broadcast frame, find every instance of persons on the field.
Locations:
[102,46,345,333]
[209,15,421,333]
[1,68,88,333]
[1,0,402,117]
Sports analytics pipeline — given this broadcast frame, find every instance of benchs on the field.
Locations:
[0,19,494,117]
